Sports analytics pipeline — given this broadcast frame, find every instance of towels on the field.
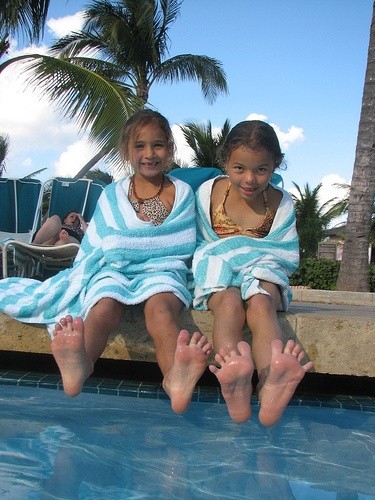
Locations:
[0,174,195,341]
[191,175,300,312]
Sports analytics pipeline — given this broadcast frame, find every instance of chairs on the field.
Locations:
[0,177,107,279]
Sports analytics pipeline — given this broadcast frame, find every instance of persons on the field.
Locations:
[194,120,314,426]
[32,211,88,246]
[51,109,212,413]
